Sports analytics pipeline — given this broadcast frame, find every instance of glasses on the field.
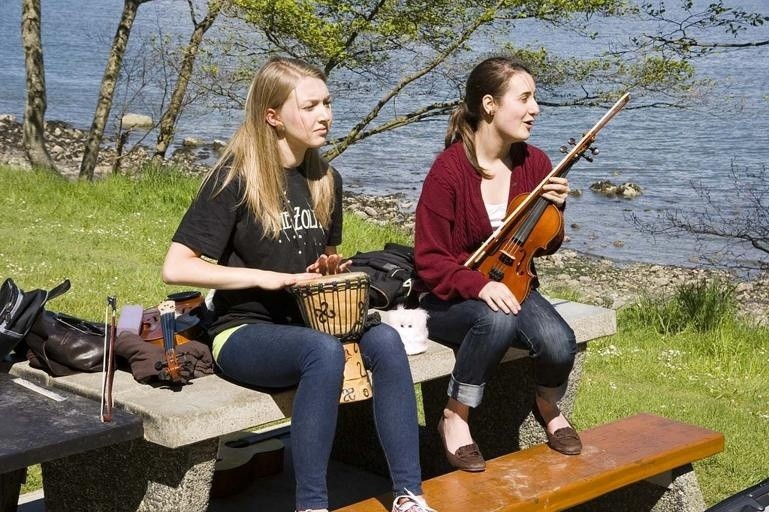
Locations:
[55,314,104,336]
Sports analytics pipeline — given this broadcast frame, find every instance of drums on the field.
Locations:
[286,271,372,405]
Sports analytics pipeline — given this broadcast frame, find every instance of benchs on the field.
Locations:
[330,415,727,511]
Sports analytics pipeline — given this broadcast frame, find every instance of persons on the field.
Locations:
[162,55,434,512]
[413,57,583,473]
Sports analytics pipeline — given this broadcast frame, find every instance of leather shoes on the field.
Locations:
[437,415,486,471]
[534,401,582,455]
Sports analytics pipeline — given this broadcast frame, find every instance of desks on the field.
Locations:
[9,293,620,511]
[0,372,144,510]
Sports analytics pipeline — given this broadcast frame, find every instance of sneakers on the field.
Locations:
[391,495,436,512]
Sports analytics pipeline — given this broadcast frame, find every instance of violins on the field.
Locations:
[141,291,207,387]
[462,133,599,305]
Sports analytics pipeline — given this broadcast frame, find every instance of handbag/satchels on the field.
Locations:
[0,278,48,362]
